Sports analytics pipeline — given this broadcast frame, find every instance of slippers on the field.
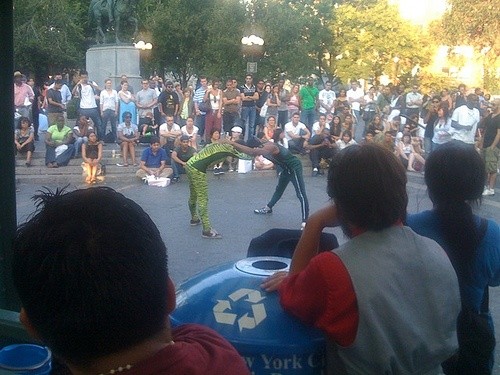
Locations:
[408,168,416,172]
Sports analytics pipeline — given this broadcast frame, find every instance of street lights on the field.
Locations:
[134,40,153,81]
[241,35,265,74]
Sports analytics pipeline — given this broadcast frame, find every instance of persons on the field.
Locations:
[260,141,462,375]
[403,140,499,375]
[13,71,500,240]
[10,186,250,375]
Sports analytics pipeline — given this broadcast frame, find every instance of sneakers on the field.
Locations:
[190,218,202,225]
[301,222,306,230]
[202,227,223,239]
[482,189,495,196]
[213,167,224,174]
[254,206,272,215]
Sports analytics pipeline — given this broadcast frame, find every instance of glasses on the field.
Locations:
[490,103,498,106]
[168,86,173,88]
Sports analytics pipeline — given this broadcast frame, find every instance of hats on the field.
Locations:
[14,71,22,77]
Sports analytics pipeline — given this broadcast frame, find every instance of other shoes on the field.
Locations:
[48,162,58,168]
[228,169,238,172]
[123,162,128,167]
[132,162,137,167]
[25,161,30,167]
[35,136,39,141]
[86,177,96,184]
[100,141,106,146]
[312,171,318,177]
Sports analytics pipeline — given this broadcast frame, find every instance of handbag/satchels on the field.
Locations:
[260,102,268,117]
[199,90,212,112]
